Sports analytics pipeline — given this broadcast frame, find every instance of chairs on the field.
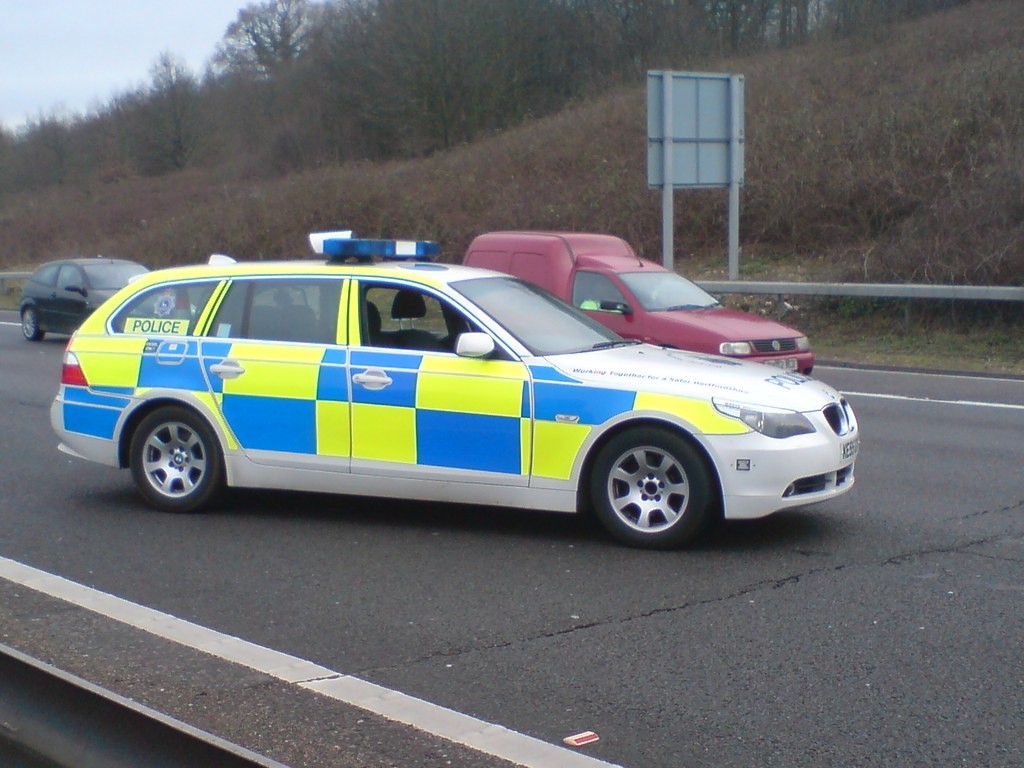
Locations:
[220,304,288,337]
[389,289,435,348]
[281,304,317,330]
[367,302,392,346]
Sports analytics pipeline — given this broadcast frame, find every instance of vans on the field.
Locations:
[464,231,815,376]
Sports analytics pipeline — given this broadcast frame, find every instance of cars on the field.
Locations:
[46,235,861,551]
[20,257,149,341]
